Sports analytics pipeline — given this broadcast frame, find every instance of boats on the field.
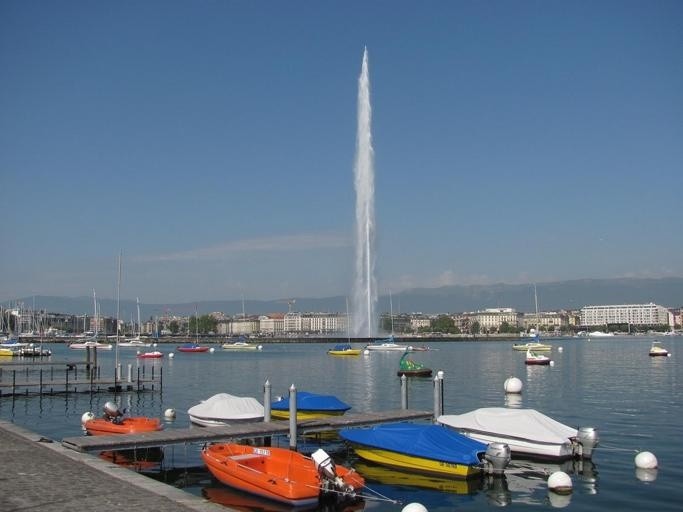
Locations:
[0,338,52,357]
[434,407,586,463]
[364,343,413,352]
[117,341,158,348]
[177,346,210,352]
[199,439,366,506]
[523,351,550,365]
[353,460,486,497]
[222,341,264,350]
[97,446,164,475]
[648,345,668,356]
[511,342,552,351]
[80,400,164,436]
[397,366,433,378]
[70,342,112,350]
[327,347,362,355]
[336,420,492,481]
[270,391,352,423]
[186,393,267,427]
[501,458,598,497]
[137,351,164,358]
